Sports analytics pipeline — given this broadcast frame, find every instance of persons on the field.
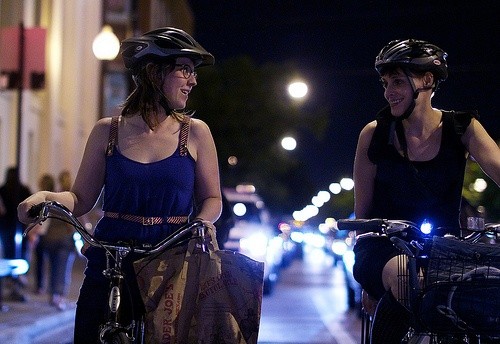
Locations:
[0,167,90,310]
[18,26,223,344]
[352,39,500,344]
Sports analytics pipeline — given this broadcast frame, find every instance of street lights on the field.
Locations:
[91,24,121,119]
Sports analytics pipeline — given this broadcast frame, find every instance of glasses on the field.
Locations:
[175,63,198,79]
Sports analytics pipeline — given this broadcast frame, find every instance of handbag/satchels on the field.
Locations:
[392,233,500,286]
[132,220,265,344]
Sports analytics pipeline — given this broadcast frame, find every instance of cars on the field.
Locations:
[272,215,364,310]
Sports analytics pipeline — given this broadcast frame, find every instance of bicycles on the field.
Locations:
[22,201,209,344]
[337,217,500,344]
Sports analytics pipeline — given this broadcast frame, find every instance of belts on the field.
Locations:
[103,210,189,225]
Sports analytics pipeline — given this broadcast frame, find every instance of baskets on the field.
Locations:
[393,244,500,336]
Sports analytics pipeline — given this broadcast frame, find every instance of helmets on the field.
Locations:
[120,26,215,74]
[375,38,448,81]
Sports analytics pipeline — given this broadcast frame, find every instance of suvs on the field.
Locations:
[221,187,285,297]
